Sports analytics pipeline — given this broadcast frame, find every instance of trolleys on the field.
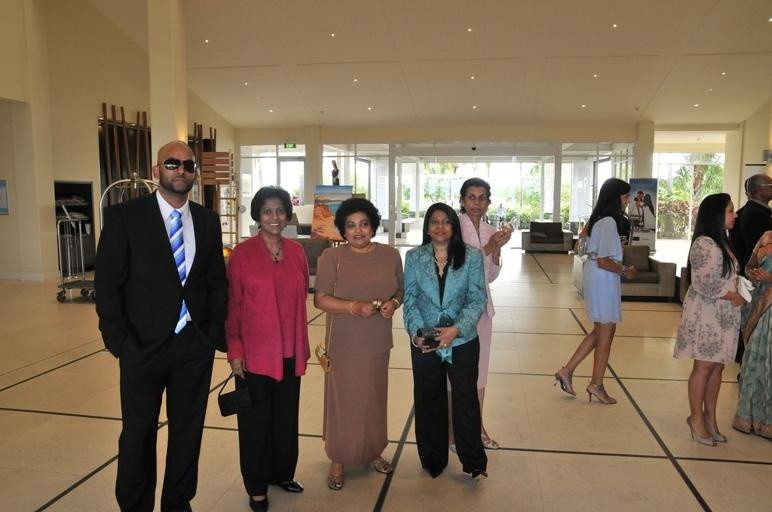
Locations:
[50,216,99,304]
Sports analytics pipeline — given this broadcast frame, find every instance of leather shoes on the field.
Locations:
[246,495,269,510]
[278,480,306,493]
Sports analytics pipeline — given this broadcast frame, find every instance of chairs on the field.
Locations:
[522,222,573,254]
[679,267,689,303]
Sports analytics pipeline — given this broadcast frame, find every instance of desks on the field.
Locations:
[394,217,417,239]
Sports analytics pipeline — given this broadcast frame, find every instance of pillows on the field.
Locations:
[531,232,547,240]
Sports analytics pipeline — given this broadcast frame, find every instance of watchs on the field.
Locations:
[621,265,630,276]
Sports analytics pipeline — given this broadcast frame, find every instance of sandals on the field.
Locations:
[482,428,500,451]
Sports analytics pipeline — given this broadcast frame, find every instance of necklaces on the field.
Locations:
[430,247,450,266]
[268,247,282,264]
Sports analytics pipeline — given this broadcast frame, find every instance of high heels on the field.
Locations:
[685,413,726,447]
[328,459,347,493]
[373,454,394,475]
[552,365,578,397]
[471,472,490,486]
[584,380,617,405]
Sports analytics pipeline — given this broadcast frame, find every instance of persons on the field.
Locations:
[633,196,655,230]
[402,202,488,480]
[555,178,637,405]
[446,178,514,452]
[225,187,311,512]
[672,173,772,447]
[94,141,230,512]
[313,198,404,490]
[637,190,654,215]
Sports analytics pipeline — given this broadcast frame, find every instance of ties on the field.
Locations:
[168,210,187,321]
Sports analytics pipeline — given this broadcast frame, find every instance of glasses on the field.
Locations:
[160,158,198,174]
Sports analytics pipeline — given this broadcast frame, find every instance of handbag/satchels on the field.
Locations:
[315,346,334,373]
[416,326,448,350]
[216,387,255,417]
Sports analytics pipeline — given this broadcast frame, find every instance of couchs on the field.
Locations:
[574,245,676,297]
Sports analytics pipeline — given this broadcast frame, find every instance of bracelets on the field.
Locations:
[411,333,420,348]
[391,298,400,309]
[348,300,363,316]
